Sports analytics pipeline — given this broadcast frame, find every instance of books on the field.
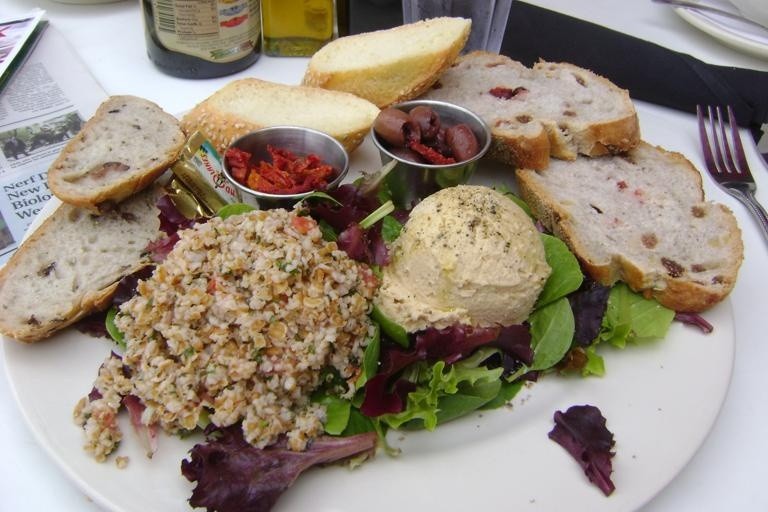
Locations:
[0,9,114,270]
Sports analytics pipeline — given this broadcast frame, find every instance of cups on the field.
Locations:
[220,125,350,210]
[369,99,493,211]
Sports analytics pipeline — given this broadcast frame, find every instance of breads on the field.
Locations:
[515,139,745,314]
[298,15,472,113]
[46,94,189,212]
[181,76,384,163]
[1,185,167,346]
[425,48,642,169]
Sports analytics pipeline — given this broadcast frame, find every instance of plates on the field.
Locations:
[669,0,767,63]
[0,129,736,512]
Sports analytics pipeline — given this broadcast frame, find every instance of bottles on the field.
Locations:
[138,0,334,80]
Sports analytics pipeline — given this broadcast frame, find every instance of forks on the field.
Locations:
[695,104,767,247]
